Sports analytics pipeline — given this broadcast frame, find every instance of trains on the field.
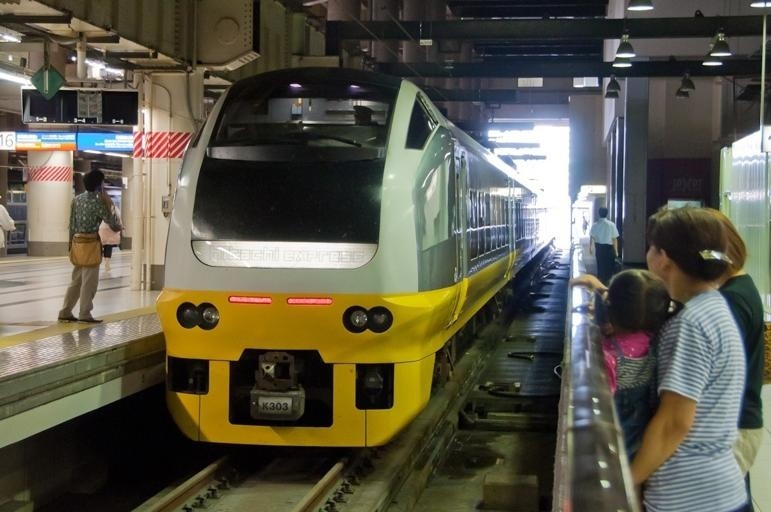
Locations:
[156,66,555,447]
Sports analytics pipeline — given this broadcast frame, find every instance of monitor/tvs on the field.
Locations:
[20,86,140,126]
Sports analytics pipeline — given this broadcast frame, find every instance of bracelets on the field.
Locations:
[598,285,608,294]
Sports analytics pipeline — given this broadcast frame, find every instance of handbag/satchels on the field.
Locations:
[70,233,102,267]
[614,257,623,273]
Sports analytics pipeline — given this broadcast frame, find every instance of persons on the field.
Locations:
[570,207,751,512]
[0,193,17,259]
[704,207,767,512]
[583,216,590,236]
[57,169,125,324]
[593,268,685,461]
[590,207,618,286]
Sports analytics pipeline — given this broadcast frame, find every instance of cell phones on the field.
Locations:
[100,180,104,192]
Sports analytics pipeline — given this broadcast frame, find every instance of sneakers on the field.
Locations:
[79,317,104,324]
[58,315,78,322]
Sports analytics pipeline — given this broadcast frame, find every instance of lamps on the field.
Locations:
[603,32,636,100]
[675,75,695,98]
[701,32,733,68]
